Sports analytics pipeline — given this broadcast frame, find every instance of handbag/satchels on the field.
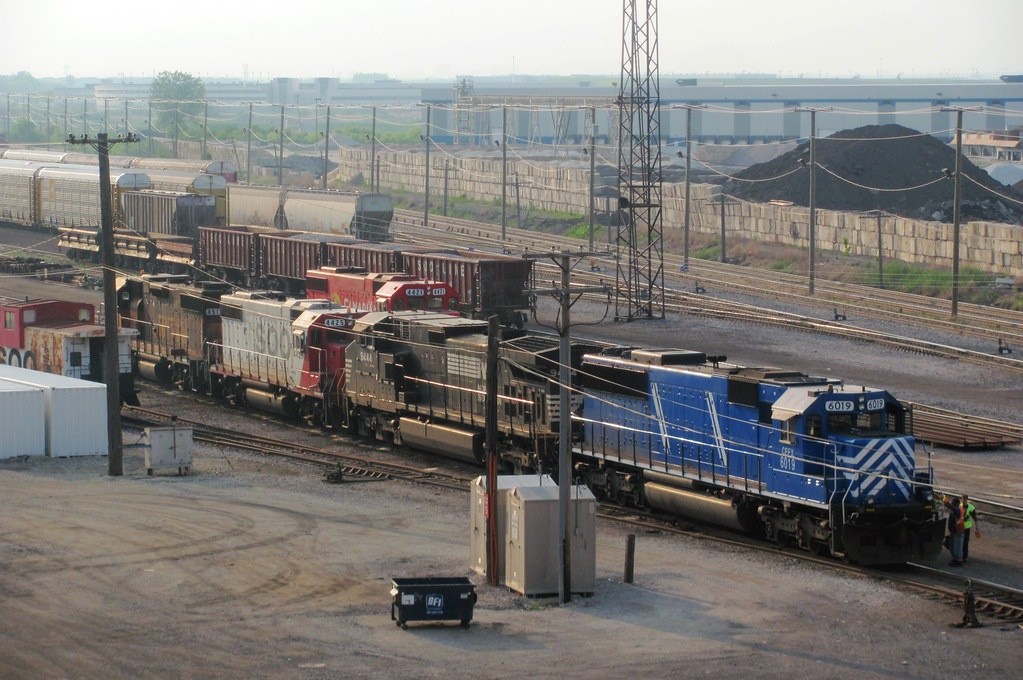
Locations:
[943,535,952,548]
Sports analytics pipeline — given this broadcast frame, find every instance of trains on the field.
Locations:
[58,225,536,328]
[0,295,140,411]
[0,150,395,243]
[97,272,947,567]
[307,265,462,319]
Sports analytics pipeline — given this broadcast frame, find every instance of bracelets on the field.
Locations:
[976,528,978,529]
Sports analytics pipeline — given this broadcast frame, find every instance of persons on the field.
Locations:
[959,495,979,562]
[937,493,964,565]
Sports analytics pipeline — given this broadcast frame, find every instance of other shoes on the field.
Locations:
[963,558,968,563]
[950,560,963,567]
